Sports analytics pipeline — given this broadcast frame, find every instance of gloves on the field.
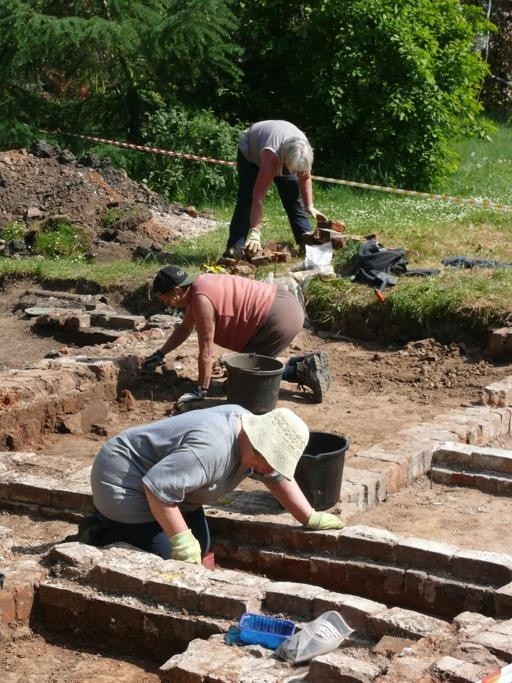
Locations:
[244,227,262,253]
[142,349,166,371]
[303,508,344,530]
[168,528,202,565]
[175,387,207,406]
[306,204,328,222]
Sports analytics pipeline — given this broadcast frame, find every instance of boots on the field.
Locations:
[296,350,330,403]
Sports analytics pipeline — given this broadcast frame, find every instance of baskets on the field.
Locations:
[238,612,296,651]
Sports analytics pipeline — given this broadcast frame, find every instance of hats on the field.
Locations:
[153,267,197,293]
[242,408,310,482]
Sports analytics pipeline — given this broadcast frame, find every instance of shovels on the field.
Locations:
[375,271,398,302]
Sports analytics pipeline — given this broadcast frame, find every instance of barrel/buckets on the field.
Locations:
[295,429,349,510]
[222,353,285,413]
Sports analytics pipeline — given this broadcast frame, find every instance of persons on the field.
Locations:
[89,404,344,565]
[144,266,331,409]
[223,120,328,259]
